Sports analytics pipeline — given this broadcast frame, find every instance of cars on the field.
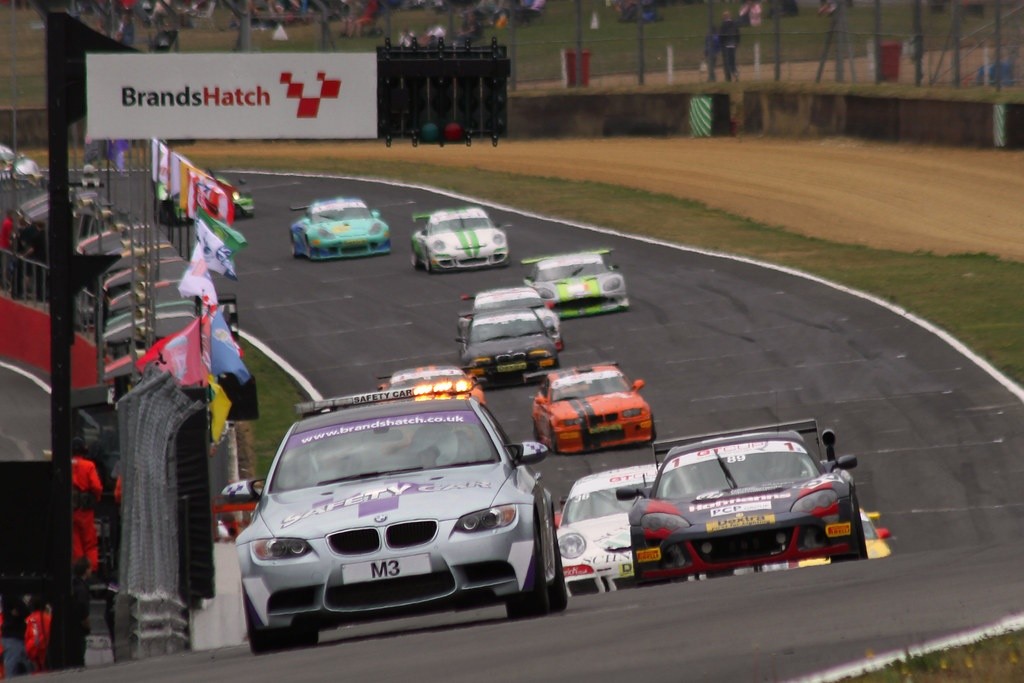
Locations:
[287,195,390,263]
[552,461,717,598]
[408,206,510,274]
[520,247,629,321]
[523,359,655,453]
[155,175,254,228]
[459,285,562,354]
[615,419,869,585]
[455,305,562,391]
[378,363,488,407]
[220,380,570,656]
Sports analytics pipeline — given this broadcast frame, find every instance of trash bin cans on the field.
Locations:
[566,50,592,87]
[880,41,902,81]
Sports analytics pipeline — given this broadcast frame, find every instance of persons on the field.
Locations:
[736,0,762,26]
[84,139,130,176]
[64,0,545,47]
[614,0,657,23]
[704,26,720,83]
[719,11,741,82]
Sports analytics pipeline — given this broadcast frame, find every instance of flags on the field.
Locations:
[149,138,274,459]
